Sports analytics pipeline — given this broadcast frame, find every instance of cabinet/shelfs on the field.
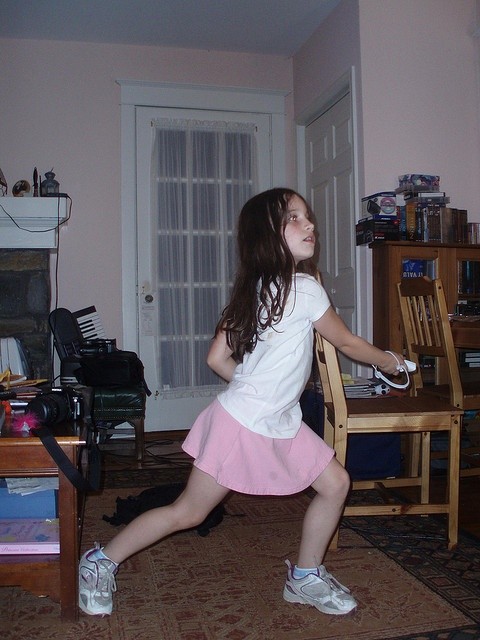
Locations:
[368,240,480,481]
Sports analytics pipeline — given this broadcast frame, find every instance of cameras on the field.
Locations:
[25,385,89,435]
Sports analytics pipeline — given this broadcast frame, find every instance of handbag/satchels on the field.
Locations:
[74,351,152,397]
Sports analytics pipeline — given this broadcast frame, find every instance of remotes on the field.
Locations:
[404,359,417,375]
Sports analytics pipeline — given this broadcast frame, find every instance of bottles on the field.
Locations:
[41,172,59,196]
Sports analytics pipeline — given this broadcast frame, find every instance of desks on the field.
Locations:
[414,320,480,385]
[2,420,91,624]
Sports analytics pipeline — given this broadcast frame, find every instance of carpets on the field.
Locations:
[0,466,479,639]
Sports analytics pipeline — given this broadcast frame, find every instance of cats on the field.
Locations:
[102,484,223,536]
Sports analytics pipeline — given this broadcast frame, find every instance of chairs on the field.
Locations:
[312,269,465,552]
[396,275,480,504]
[48,308,146,470]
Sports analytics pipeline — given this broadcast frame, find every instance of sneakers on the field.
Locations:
[79,540,117,616]
[282,559,357,614]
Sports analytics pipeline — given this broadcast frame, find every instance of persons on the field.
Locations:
[77,187,408,615]
